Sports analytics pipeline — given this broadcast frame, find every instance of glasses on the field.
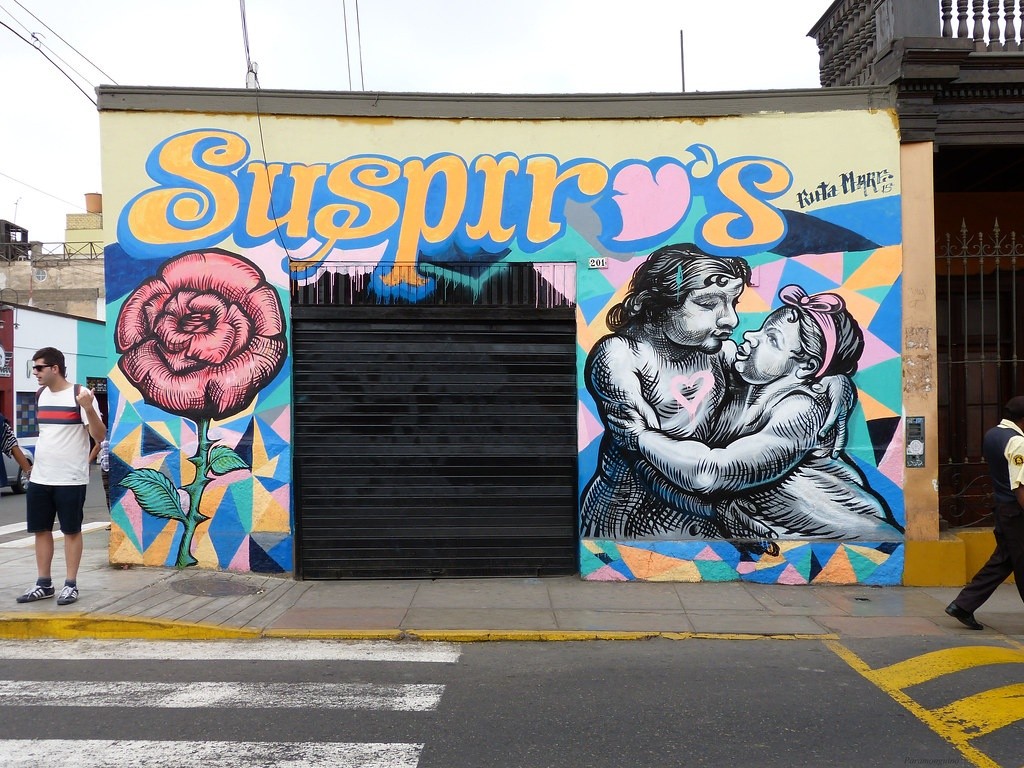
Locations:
[32,364,53,372]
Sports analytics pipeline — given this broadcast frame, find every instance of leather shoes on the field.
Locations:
[945,602,984,630]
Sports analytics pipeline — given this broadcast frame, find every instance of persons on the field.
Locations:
[945,396,1024,630]
[90,429,111,530]
[0,414,32,488]
[17,348,106,605]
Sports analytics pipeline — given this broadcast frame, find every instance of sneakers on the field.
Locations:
[56,585,79,605]
[16,585,55,604]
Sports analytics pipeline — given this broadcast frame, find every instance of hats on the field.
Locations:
[1006,395,1024,420]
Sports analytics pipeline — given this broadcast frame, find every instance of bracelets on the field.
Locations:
[24,471,31,478]
